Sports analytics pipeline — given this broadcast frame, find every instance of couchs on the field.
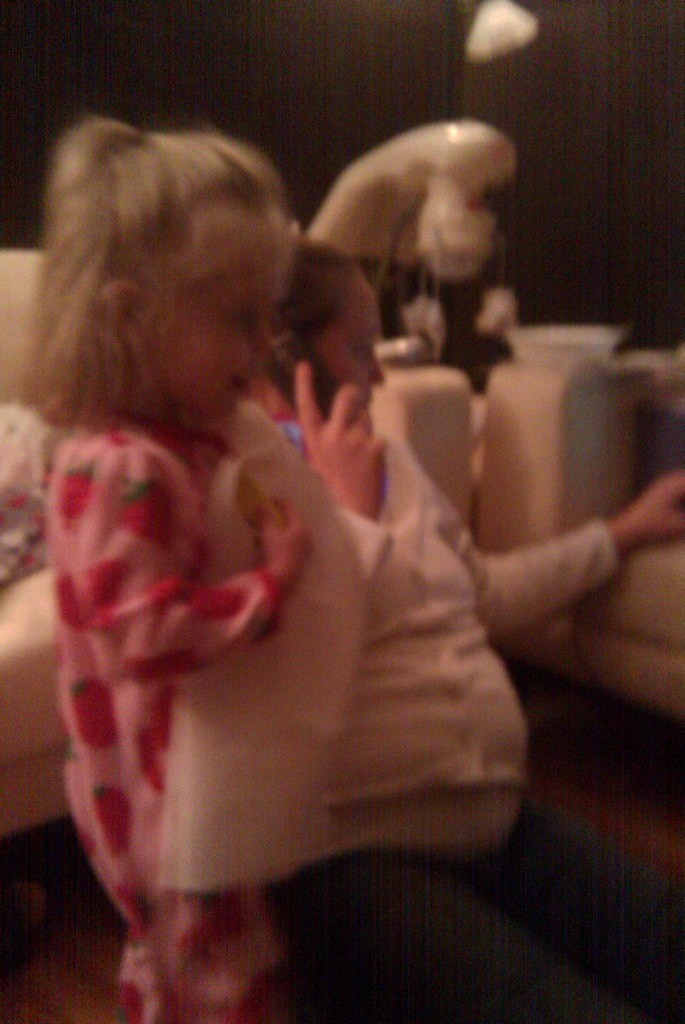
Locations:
[478,339,683,723]
[0,246,480,839]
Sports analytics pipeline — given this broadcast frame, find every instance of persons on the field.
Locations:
[13,113,313,1024]
[253,225,684,1022]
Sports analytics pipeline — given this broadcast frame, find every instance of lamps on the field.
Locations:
[436,3,534,128]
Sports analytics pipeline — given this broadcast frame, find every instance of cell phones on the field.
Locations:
[269,326,338,422]
